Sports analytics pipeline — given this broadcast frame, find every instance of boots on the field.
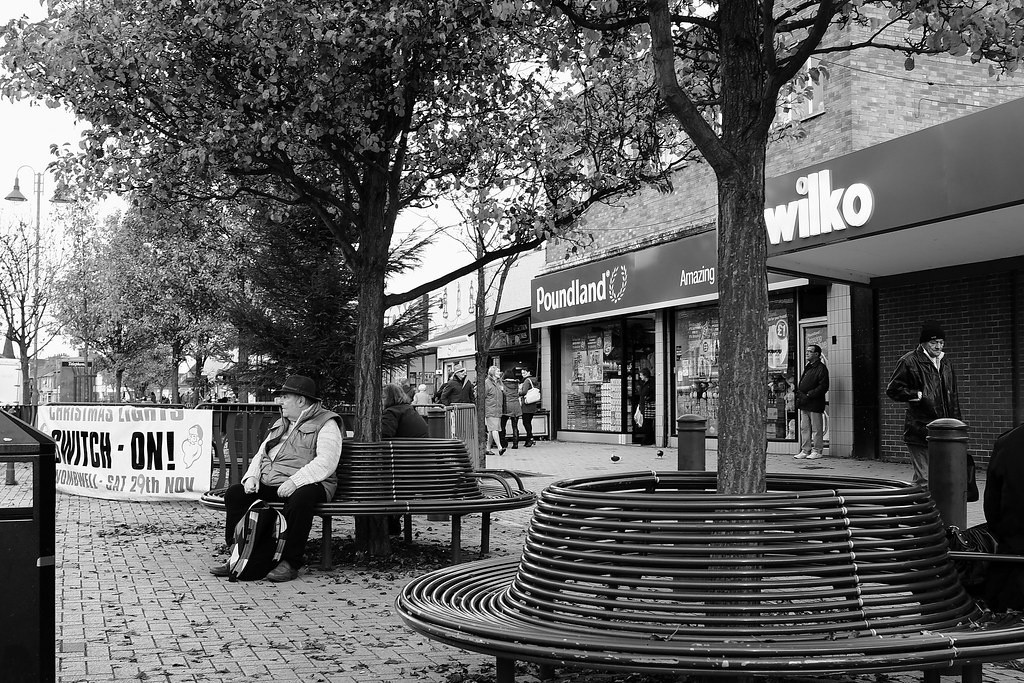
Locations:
[512,429,519,448]
[500,430,508,448]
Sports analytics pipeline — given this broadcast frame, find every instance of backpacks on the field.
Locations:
[229,499,288,581]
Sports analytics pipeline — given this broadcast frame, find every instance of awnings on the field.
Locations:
[417,308,532,348]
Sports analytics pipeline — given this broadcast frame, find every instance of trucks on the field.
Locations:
[1,358,30,406]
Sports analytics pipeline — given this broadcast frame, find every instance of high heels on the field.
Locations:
[526,440,535,447]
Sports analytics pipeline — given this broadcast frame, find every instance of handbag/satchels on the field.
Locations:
[967,454,978,502]
[947,522,999,578]
[634,405,643,427]
[524,378,541,404]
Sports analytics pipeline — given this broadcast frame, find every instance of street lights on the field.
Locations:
[4,160,76,404]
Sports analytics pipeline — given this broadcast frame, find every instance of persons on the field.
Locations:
[500,370,522,449]
[432,366,477,406]
[638,367,655,445]
[518,367,539,447]
[886,320,961,484]
[210,375,345,581]
[381,383,429,438]
[484,365,506,455]
[400,378,415,401]
[410,384,432,423]
[983,422,1024,611]
[150,390,195,404]
[794,345,829,459]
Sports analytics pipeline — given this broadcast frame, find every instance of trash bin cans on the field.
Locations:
[0,408,56,683]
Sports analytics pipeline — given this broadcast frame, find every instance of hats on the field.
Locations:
[271,375,322,402]
[418,384,426,391]
[454,364,465,373]
[919,320,945,343]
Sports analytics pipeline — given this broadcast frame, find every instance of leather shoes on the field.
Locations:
[267,561,297,581]
[210,557,231,575]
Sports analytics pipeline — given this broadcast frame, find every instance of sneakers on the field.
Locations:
[806,452,823,459]
[794,449,810,459]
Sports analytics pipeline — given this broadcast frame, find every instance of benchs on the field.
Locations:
[393,469,1024,683]
[200,438,538,571]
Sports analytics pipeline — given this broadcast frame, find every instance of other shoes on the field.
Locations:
[499,448,506,456]
[486,451,495,455]
